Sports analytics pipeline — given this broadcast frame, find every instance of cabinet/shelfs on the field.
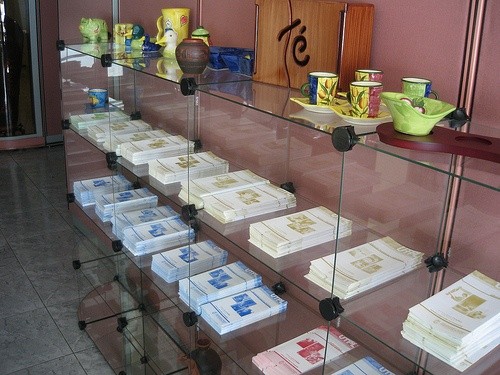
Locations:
[58,44,500,375]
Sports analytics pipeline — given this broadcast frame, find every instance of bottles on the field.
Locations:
[188,338,222,375]
[175,38,209,74]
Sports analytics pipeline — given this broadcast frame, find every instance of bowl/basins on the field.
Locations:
[380,91,457,137]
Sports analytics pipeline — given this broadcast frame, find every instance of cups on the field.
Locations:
[191,26,211,47]
[156,8,189,58]
[353,68,383,83]
[349,81,383,118]
[88,88,109,107]
[401,76,439,101]
[301,71,338,107]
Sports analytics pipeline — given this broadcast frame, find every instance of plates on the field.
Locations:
[289,97,350,114]
[336,91,350,99]
[330,102,393,125]
